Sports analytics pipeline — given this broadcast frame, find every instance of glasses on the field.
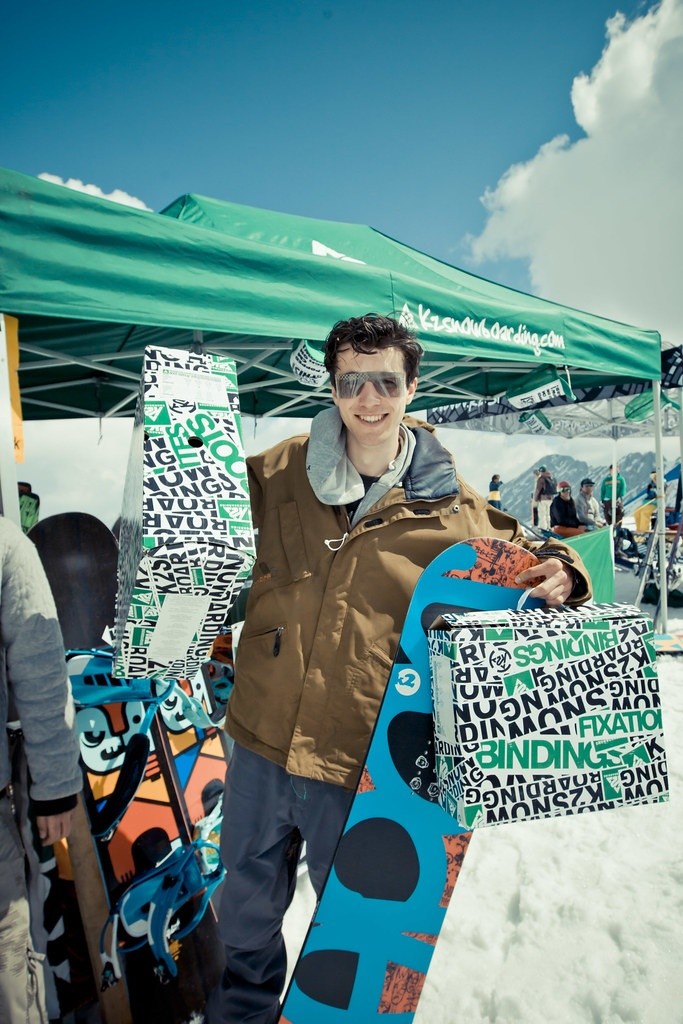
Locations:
[334,372,406,400]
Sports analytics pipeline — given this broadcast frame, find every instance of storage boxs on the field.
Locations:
[427,607,668,830]
[112,345,254,684]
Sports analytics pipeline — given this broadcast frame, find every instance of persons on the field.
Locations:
[646,471,657,494]
[0,509,82,1024]
[211,311,592,1024]
[488,473,504,510]
[549,480,587,535]
[531,466,552,530]
[575,478,606,528]
[600,465,626,527]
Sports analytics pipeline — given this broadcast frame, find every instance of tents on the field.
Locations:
[0,166,669,633]
[425,339,682,614]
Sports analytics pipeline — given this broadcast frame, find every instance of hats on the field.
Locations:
[581,479,595,485]
[538,466,546,472]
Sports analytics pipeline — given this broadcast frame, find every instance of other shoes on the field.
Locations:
[632,562,640,575]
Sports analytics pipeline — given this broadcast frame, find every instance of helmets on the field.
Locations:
[557,481,571,492]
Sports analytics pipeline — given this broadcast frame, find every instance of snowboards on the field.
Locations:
[270,536,549,1024]
[16,511,226,1024]
